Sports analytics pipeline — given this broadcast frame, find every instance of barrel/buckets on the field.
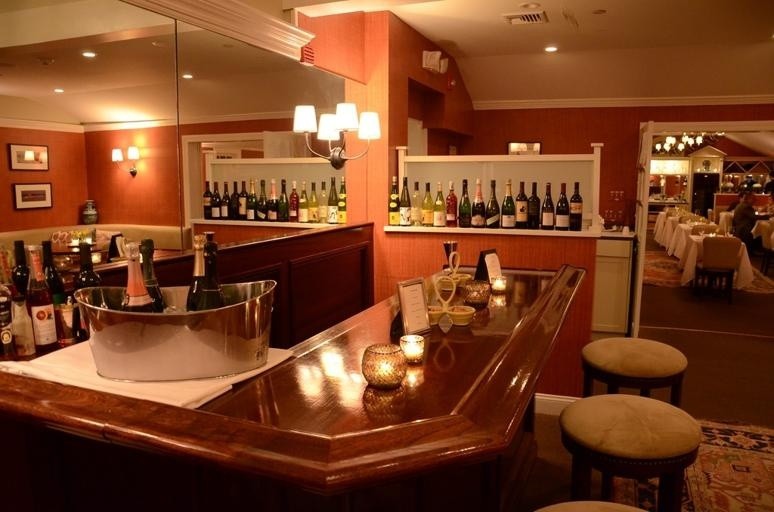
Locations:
[72,279,278,383]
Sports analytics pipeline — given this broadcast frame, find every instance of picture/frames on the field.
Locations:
[13,182,53,211]
[7,143,49,172]
[397,278,431,336]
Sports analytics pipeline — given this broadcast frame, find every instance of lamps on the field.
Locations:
[332,102,382,170]
[111,147,138,177]
[293,104,340,168]
[654,133,704,153]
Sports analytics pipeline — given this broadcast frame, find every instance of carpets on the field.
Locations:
[587,416,774,512]
[642,225,774,294]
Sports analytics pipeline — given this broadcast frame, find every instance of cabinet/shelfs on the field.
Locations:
[590,236,634,334]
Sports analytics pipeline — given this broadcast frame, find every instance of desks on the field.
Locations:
[47,242,103,269]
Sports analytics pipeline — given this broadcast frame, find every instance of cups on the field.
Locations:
[426,273,510,326]
[362,381,405,425]
[427,323,475,344]
[362,333,426,389]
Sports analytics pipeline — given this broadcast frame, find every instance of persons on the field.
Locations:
[727,192,746,213]
[765,171,774,200]
[733,193,771,250]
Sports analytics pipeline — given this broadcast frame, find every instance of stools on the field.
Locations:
[533,498,650,512]
[581,337,688,483]
[558,392,701,511]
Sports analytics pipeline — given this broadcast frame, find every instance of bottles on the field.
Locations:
[387,171,584,231]
[0,230,221,362]
[512,274,528,323]
[201,176,347,226]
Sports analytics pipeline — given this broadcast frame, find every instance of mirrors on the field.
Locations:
[0,0,369,290]
[505,139,542,155]
